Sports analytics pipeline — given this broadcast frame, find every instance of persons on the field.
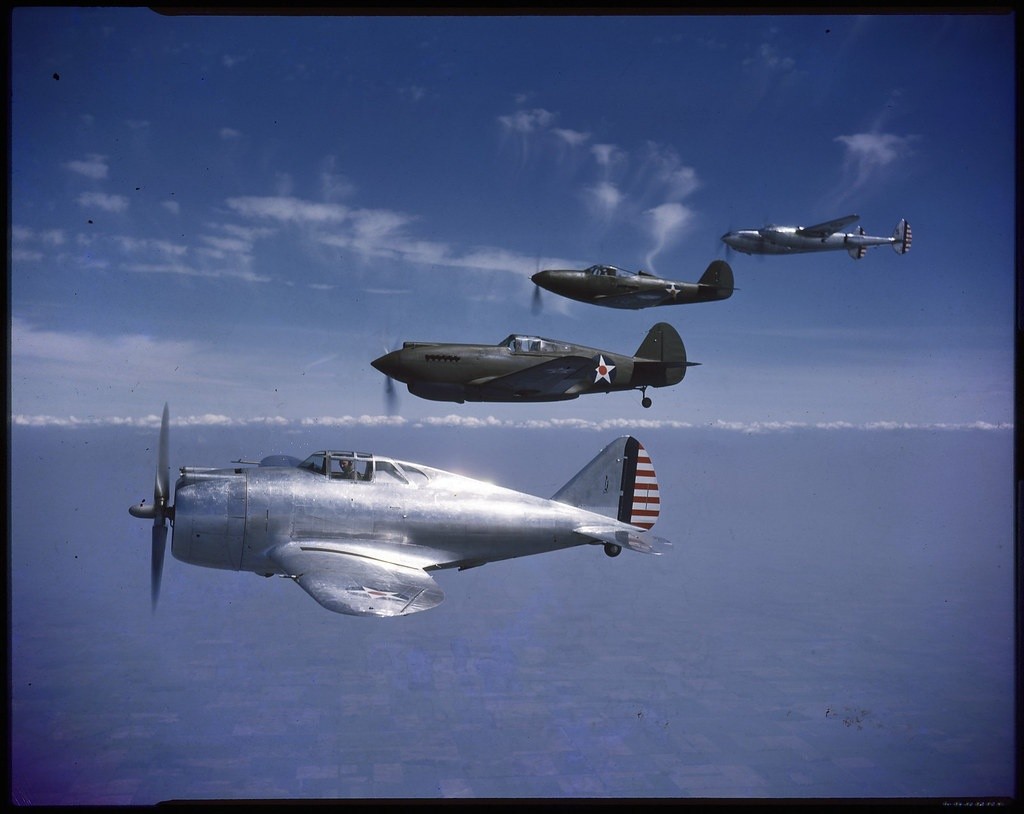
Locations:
[339,458,363,480]
[513,339,523,353]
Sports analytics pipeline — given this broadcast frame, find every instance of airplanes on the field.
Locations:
[758,214,913,257]
[721,228,823,256]
[371,321,704,420]
[527,259,742,318]
[126,399,674,619]
